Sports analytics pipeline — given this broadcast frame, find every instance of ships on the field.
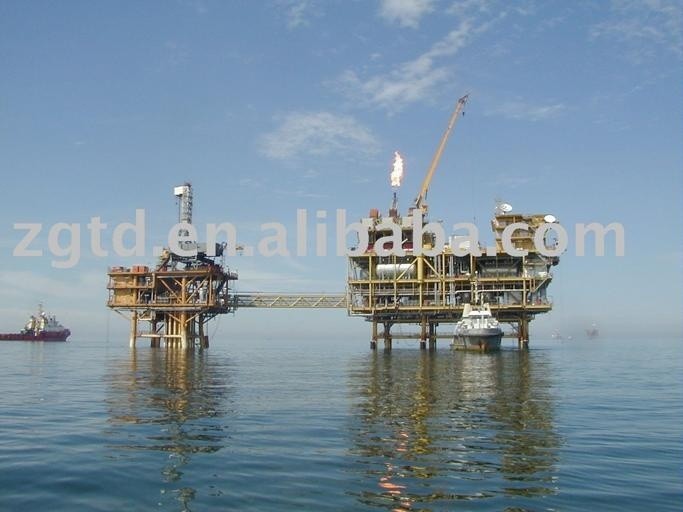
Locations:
[0,301,71,342]
[450,301,503,352]
